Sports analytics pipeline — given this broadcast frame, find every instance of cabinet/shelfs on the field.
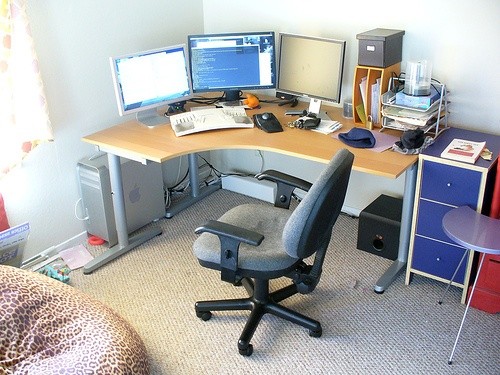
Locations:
[379,77,451,139]
[352,63,402,130]
[405,127,500,305]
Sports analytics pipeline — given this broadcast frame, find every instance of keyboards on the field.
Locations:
[169,106,254,137]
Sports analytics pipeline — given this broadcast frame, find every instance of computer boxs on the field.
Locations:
[77,152,166,248]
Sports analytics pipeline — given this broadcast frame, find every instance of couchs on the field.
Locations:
[0,262,150,375]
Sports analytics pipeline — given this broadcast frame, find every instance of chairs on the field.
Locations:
[193,149,355,356]
[467,156,500,314]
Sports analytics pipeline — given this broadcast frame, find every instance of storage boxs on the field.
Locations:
[356,28,405,67]
[0,222,30,268]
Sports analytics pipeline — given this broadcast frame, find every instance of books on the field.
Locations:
[440,138,488,164]
[380,102,452,132]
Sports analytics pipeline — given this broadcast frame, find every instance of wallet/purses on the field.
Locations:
[304,118,321,129]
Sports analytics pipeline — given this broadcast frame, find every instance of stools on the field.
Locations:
[438,205,500,365]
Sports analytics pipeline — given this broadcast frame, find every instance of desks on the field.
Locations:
[81,92,447,294]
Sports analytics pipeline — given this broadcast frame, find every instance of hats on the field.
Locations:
[338,127,375,148]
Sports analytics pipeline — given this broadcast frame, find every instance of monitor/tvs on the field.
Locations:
[188,31,276,109]
[276,32,346,120]
[109,43,194,128]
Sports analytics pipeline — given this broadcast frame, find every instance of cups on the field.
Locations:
[343,101,352,117]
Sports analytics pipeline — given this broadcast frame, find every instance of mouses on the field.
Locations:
[262,112,272,120]
